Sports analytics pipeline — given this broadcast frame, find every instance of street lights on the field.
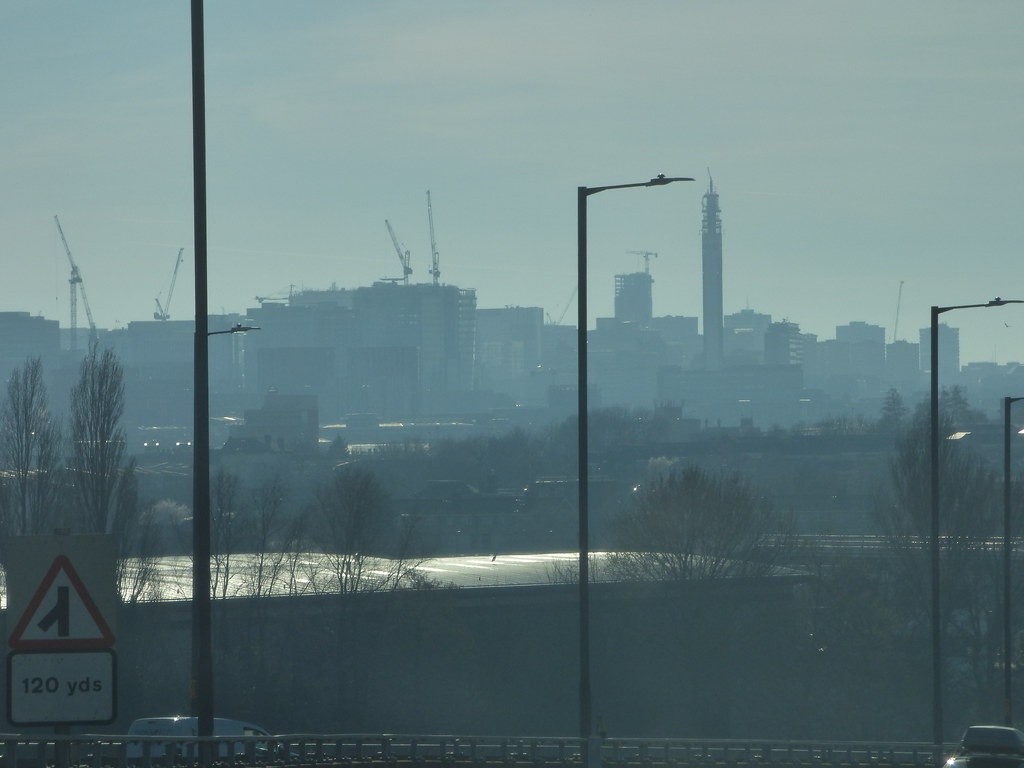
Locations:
[1001,395,1024,734]
[574,172,696,767]
[928,298,1024,768]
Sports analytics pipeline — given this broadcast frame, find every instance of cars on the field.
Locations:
[144,439,160,452]
[174,439,193,449]
[124,716,285,768]
[942,725,1024,768]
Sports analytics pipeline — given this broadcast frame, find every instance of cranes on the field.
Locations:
[625,249,657,274]
[384,219,413,285]
[53,214,101,350]
[426,190,440,283]
[153,248,183,320]
